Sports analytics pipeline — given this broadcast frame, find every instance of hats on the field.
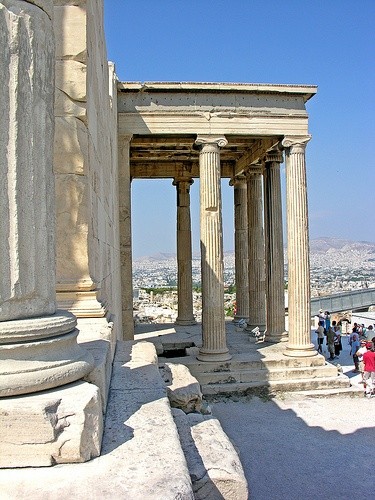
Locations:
[365,343,372,348]
[361,341,366,344]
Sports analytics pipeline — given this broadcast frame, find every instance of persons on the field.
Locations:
[348,322,375,398]
[325,321,342,361]
[318,309,325,327]
[324,311,331,332]
[315,321,324,354]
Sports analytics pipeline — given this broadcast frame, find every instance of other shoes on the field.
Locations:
[328,358,334,361]
[352,369,359,373]
[335,355,339,359]
